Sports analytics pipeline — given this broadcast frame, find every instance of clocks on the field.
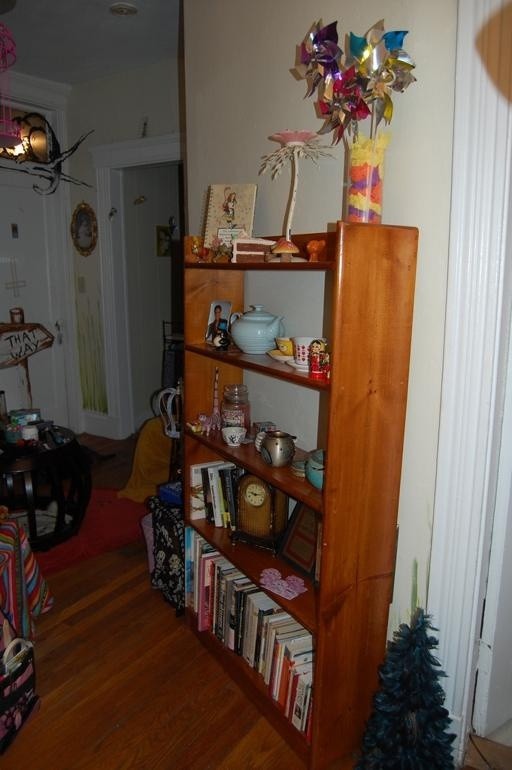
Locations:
[231,472,289,558]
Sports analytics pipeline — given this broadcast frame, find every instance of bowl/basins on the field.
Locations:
[275,335,295,354]
[222,426,246,446]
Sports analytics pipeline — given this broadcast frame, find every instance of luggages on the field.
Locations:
[150,501,185,617]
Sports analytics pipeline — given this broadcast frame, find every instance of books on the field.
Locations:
[182,525,314,746]
[187,459,245,532]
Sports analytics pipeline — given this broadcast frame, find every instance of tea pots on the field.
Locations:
[230,303,283,355]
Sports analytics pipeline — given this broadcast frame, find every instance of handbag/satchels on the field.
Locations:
[0,637,38,756]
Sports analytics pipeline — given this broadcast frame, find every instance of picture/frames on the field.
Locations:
[70,200,99,259]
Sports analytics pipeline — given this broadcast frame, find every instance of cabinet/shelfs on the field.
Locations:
[183,222,418,769]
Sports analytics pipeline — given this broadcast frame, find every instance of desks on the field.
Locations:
[0,425,92,551]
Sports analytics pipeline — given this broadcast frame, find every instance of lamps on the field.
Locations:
[111,4,136,16]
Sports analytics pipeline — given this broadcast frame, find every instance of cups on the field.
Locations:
[294,336,323,364]
[254,432,267,452]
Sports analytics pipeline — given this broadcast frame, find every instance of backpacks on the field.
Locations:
[123,415,174,502]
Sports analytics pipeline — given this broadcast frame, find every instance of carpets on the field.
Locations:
[33,487,151,581]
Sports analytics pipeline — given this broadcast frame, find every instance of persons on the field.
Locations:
[205,305,227,343]
[223,186,236,229]
[76,218,91,248]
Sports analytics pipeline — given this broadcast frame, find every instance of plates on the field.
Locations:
[286,359,319,371]
[269,349,293,362]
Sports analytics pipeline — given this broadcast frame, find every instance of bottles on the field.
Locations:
[221,385,251,436]
